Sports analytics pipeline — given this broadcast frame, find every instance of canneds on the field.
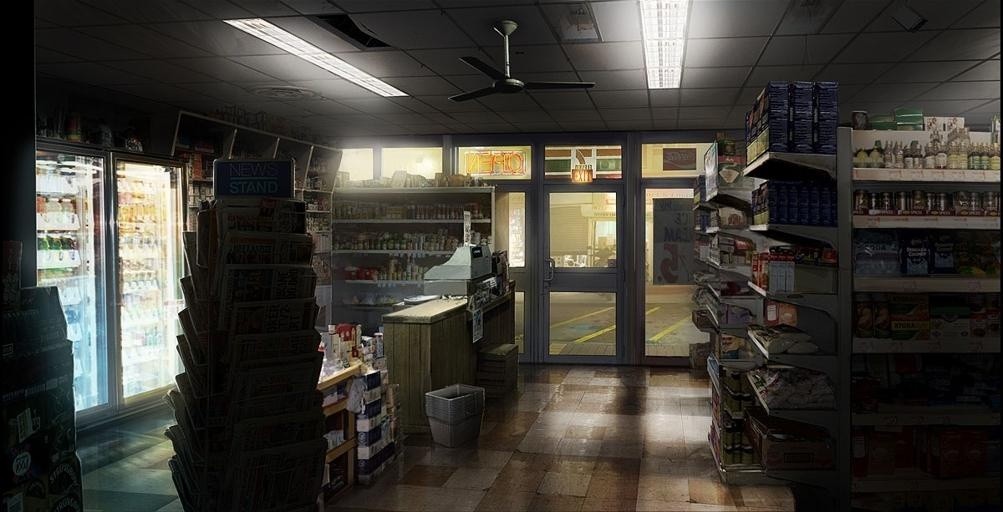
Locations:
[852,187,1002,217]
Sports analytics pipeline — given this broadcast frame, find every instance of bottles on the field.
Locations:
[303,194,328,211]
[852,189,999,214]
[335,228,491,250]
[35,148,170,412]
[853,139,1001,170]
[304,213,329,230]
[343,258,428,281]
[334,199,485,219]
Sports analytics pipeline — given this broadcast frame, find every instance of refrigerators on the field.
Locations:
[6,140,188,420]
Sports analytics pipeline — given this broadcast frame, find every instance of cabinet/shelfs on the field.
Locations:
[313,357,362,507]
[329,185,510,329]
[164,109,344,331]
[692,122,1003,509]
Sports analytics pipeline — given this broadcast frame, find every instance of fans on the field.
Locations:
[446,18,596,104]
